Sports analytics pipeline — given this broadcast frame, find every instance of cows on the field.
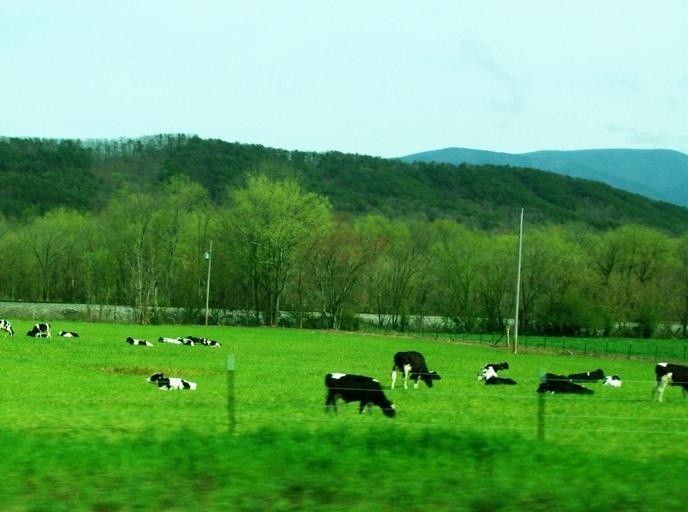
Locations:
[126,337,153,346]
[146,371,198,391]
[58,330,79,337]
[651,361,688,403]
[389,350,442,391]
[26,322,53,337]
[158,334,222,347]
[0,319,15,336]
[535,368,622,394]
[477,362,517,385]
[322,371,397,418]
